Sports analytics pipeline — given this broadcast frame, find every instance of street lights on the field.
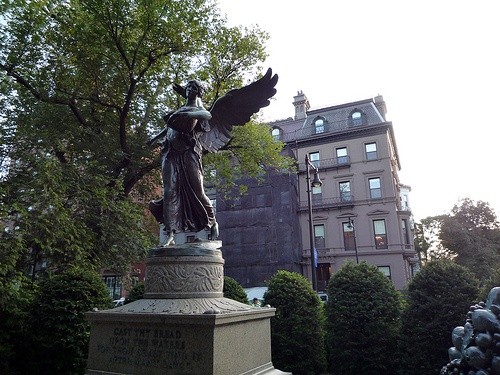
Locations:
[305,153,322,291]
[347,217,358,265]
